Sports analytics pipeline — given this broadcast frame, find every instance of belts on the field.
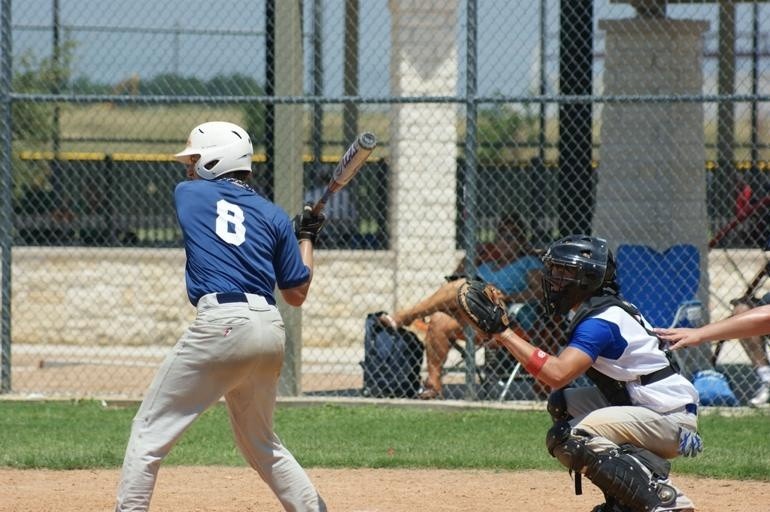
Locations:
[217,294,276,305]
[684,404,699,415]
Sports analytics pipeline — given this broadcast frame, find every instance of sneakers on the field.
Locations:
[652,491,695,511]
[749,381,769,407]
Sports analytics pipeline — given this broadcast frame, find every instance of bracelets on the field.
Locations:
[499,330,514,345]
[525,349,549,377]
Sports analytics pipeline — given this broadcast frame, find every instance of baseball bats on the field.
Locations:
[313,134,376,216]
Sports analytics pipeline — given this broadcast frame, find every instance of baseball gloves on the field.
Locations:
[458,280,510,334]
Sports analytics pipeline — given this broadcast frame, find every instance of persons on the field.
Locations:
[111,119,329,512]
[730,291,770,406]
[456,232,705,512]
[380,209,545,399]
[650,303,769,351]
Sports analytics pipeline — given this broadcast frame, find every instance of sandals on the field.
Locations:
[380,315,398,329]
[421,386,443,400]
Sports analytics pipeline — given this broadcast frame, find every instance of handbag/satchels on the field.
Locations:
[694,371,735,405]
[364,310,422,399]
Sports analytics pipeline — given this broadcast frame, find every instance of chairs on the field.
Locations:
[609,244,704,381]
[415,245,564,403]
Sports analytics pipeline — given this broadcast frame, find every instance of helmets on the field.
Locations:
[541,236,614,316]
[173,122,253,179]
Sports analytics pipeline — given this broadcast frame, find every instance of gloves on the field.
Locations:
[676,426,702,459]
[291,202,326,237]
